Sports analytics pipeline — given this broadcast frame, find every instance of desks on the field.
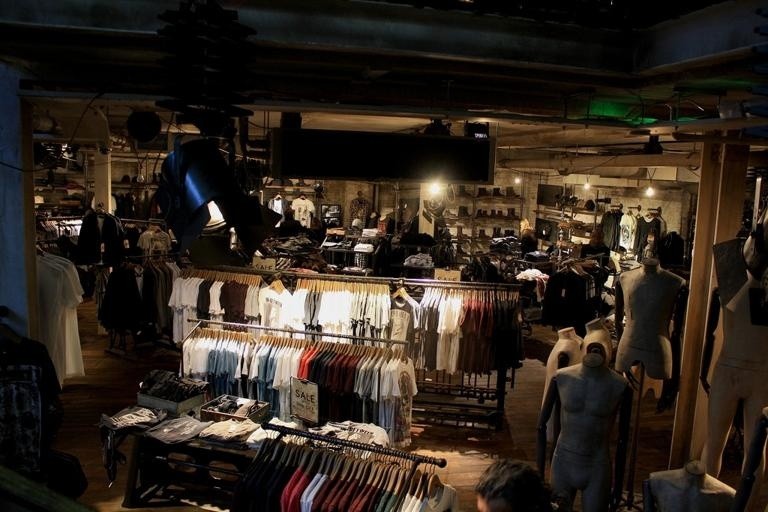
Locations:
[122,427,258,504]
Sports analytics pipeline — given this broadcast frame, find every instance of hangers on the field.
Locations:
[34,240,73,272]
[184,318,413,372]
[272,190,308,201]
[422,280,520,309]
[237,423,456,502]
[608,204,662,219]
[275,254,302,269]
[112,220,170,238]
[114,253,193,273]
[175,263,422,305]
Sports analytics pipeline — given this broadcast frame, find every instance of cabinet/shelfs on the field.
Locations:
[557,184,611,271]
[532,204,561,257]
[456,184,524,265]
[94,100,178,221]
[21,96,112,254]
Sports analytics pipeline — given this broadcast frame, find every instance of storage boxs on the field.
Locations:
[199,394,272,422]
[134,392,205,413]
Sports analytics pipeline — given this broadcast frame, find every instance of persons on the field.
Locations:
[700,225,768,511]
[472,456,555,512]
[536,257,692,511]
